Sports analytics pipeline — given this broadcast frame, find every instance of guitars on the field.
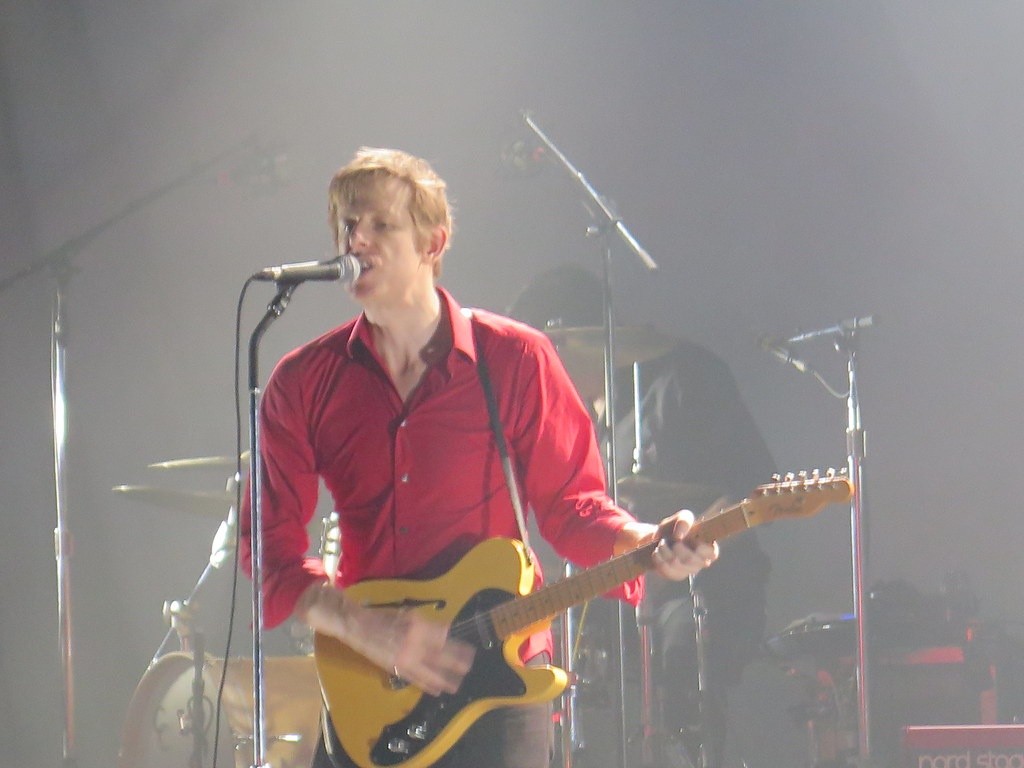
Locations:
[313,468,856,767]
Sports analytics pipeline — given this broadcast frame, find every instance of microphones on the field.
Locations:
[752,331,811,373]
[260,254,360,287]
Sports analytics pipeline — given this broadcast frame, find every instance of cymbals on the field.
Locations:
[112,485,236,510]
[146,456,254,470]
[539,324,672,364]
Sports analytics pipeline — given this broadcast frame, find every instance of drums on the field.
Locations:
[121,651,324,767]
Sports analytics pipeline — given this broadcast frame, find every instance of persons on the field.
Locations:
[505,265,777,768]
[239,145,720,767]
